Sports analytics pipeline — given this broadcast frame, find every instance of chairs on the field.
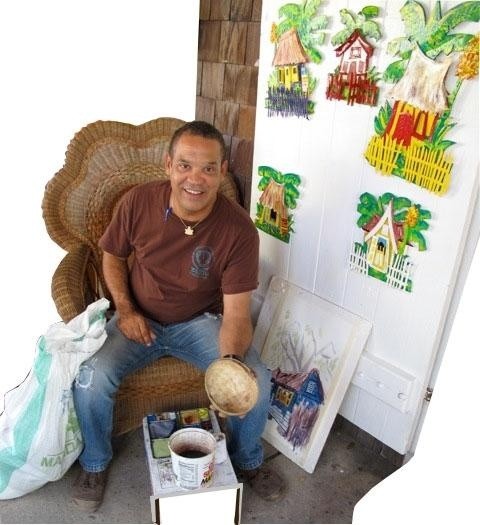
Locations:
[41,116,243,439]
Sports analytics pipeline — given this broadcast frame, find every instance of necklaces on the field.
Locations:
[171,192,216,237]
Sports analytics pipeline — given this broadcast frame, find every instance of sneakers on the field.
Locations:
[72,461,111,512]
[239,463,285,502]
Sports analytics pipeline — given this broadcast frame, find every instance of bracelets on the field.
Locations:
[221,354,243,363]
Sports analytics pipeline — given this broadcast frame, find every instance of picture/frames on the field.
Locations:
[245,273,374,474]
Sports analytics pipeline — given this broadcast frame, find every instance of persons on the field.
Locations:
[70,118,287,513]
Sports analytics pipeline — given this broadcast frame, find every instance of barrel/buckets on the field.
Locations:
[167,428,216,489]
